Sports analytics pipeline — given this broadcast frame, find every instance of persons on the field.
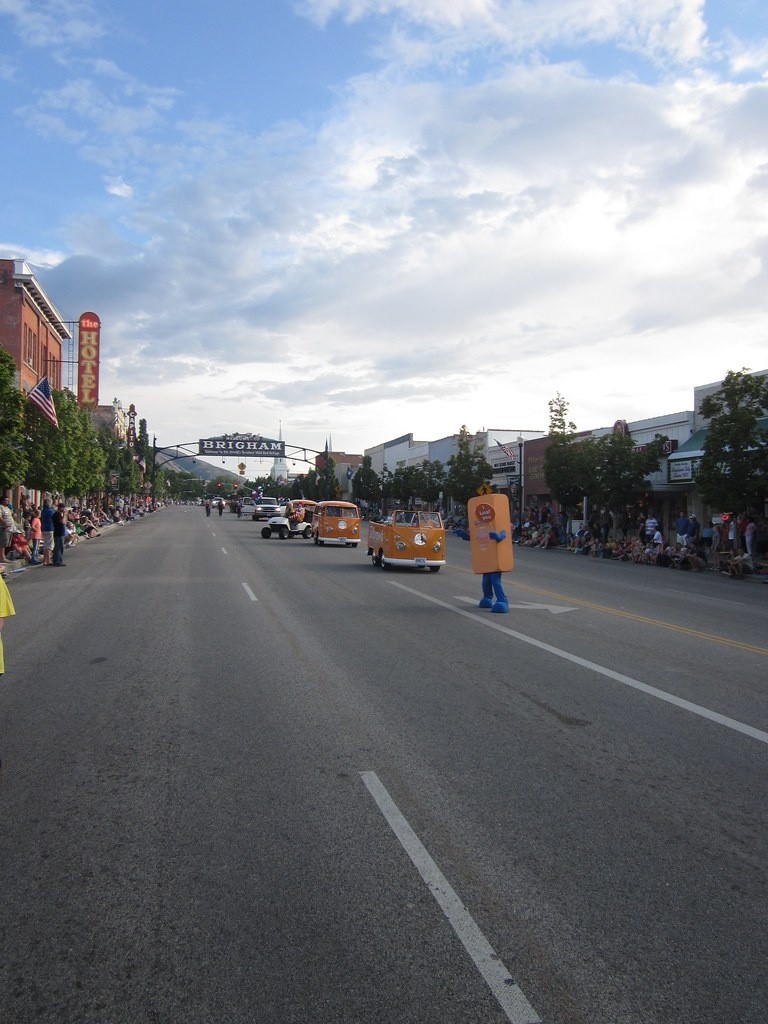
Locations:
[51,503,67,566]
[452,493,514,612]
[0,496,15,563]
[175,498,205,507]
[710,525,720,572]
[206,499,210,516]
[41,499,55,566]
[675,511,689,545]
[644,513,658,545]
[0,573,16,766]
[353,497,566,549]
[218,501,224,515]
[284,502,306,529]
[568,508,755,579]
[10,490,168,563]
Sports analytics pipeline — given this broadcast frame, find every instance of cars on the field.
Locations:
[367,510,447,572]
[212,497,227,508]
[284,499,361,547]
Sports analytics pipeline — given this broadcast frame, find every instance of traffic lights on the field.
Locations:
[234,483,238,490]
[217,482,222,488]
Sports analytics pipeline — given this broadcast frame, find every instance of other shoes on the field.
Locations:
[541,546,548,549]
[2,559,11,563]
[535,545,542,548]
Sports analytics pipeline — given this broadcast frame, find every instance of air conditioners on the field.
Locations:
[28,358,32,367]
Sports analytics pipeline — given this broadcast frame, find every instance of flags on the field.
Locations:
[139,456,146,473]
[25,373,63,431]
[166,478,171,488]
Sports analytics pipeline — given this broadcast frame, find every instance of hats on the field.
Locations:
[13,530,22,534]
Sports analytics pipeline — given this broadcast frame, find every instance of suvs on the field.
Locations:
[241,495,290,521]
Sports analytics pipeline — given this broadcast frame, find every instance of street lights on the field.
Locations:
[517,433,526,537]
